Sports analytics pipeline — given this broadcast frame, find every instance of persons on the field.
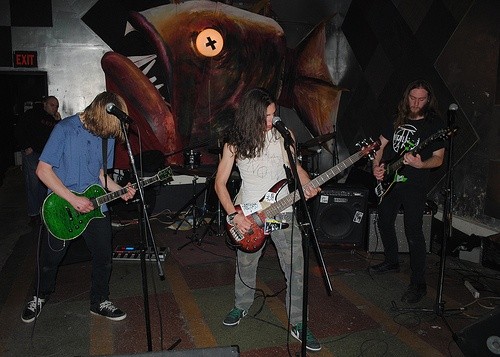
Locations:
[368,77,447,303]
[21,91,136,323]
[19,96,62,216]
[214,88,322,351]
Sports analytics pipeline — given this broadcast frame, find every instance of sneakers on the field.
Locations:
[291,322,321,351]
[21,295,48,323]
[222,307,248,325]
[90,300,127,321]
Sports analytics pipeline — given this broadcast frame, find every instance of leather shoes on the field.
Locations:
[401,283,427,303]
[371,261,399,272]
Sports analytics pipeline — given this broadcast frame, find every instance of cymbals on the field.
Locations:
[208,148,223,154]
[301,133,335,147]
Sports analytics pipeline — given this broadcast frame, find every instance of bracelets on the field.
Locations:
[228,212,238,220]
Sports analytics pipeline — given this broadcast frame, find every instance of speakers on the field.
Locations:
[114,345,240,357]
[312,188,370,250]
[455,304,500,357]
[368,207,433,254]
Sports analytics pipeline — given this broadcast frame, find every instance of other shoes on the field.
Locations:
[32,214,40,224]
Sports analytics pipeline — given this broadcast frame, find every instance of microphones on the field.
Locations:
[106,103,133,124]
[448,103,459,131]
[273,117,294,144]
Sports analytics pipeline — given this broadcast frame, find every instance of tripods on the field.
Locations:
[174,148,225,251]
[392,130,465,340]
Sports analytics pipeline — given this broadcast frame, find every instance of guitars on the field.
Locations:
[374,127,449,199]
[225,137,382,254]
[42,166,174,241]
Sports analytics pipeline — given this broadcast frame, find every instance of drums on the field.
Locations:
[188,148,218,177]
[206,176,241,214]
